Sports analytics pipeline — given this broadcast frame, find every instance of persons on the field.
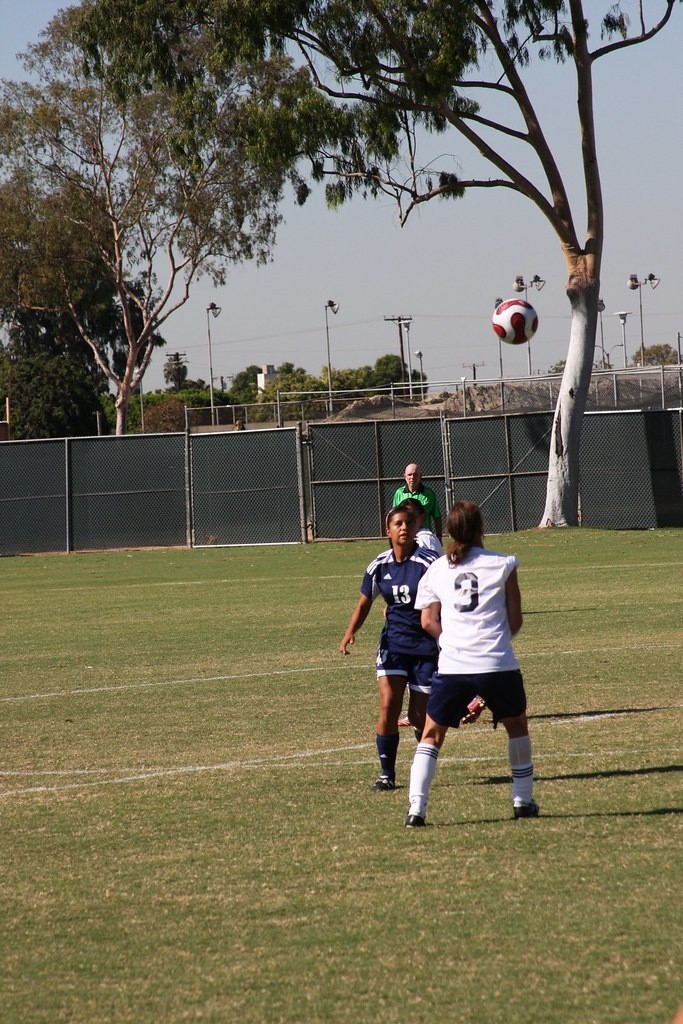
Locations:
[391,463,443,548]
[339,507,439,789]
[384,498,486,727]
[232,419,245,430]
[403,500,539,827]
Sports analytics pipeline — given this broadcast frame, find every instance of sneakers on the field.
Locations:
[372,774,395,790]
[407,714,425,743]
[405,811,425,829]
[513,798,539,819]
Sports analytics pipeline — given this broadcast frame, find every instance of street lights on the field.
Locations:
[206,301,222,425]
[595,343,623,371]
[598,298,604,370]
[627,273,660,368]
[325,299,340,412]
[414,351,424,403]
[613,311,634,369]
[513,274,546,377]
[400,320,413,400]
[494,297,503,379]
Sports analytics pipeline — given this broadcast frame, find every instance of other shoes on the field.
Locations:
[461,697,485,725]
[397,716,410,726]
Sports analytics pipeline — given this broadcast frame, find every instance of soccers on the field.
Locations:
[491,297,540,345]
